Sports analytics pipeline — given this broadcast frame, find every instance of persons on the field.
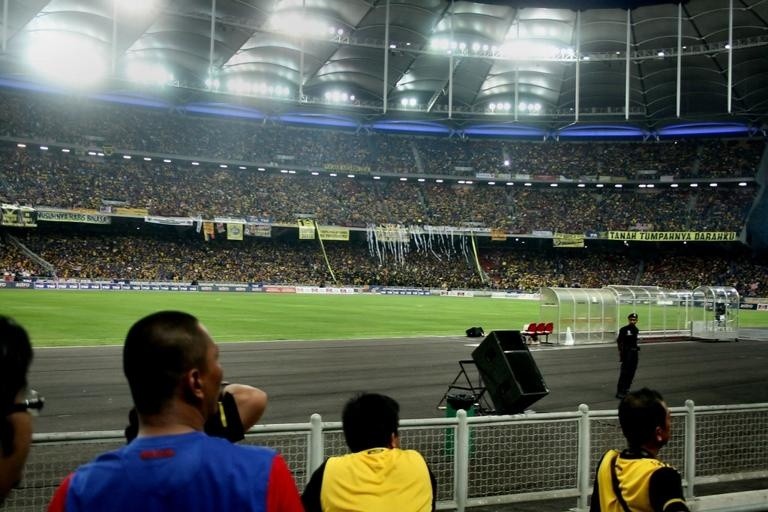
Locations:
[590,388,690,512]
[0,315,46,510]
[0,85,768,307]
[48,310,303,512]
[300,394,435,512]
[615,312,641,399]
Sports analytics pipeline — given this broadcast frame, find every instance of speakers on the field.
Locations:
[471,331,549,415]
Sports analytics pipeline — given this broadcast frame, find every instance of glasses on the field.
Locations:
[3,390,45,416]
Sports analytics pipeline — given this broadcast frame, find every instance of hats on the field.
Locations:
[628,313,638,318]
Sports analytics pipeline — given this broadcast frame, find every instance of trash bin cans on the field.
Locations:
[444,394,474,462]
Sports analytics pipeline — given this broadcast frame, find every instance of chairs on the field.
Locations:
[520,322,553,346]
